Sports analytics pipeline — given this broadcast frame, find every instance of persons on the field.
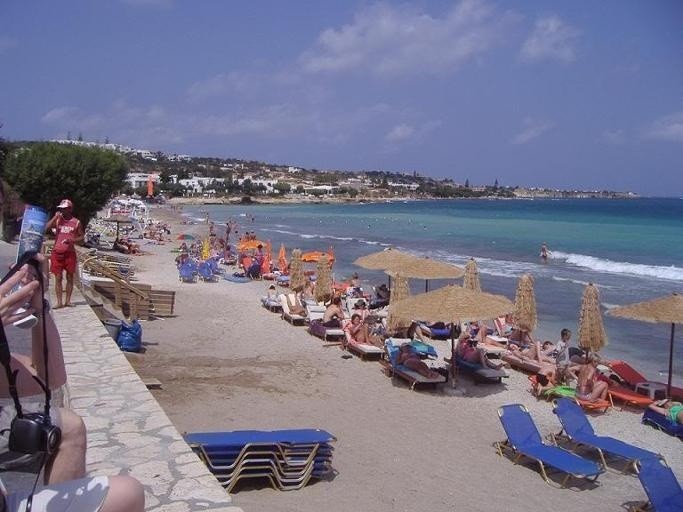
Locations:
[0,253,146,510]
[175,212,264,280]
[92,212,171,255]
[45,198,85,309]
[268,272,683,425]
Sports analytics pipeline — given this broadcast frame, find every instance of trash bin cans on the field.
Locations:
[103,318,122,341]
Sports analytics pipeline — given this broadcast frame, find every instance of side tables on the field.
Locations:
[636,382,667,398]
[423,359,448,381]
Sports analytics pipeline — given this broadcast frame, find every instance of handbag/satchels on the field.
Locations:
[55,250,66,261]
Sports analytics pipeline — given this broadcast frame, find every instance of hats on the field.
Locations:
[56,199,72,209]
[354,299,368,307]
[400,342,414,349]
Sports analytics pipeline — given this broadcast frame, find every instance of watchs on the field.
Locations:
[32,297,51,318]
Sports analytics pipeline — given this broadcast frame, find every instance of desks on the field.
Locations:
[486,335,509,346]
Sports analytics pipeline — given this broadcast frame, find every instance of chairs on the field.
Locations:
[531,375,607,412]
[277,276,289,285]
[597,371,652,412]
[307,306,344,342]
[181,427,335,491]
[449,339,508,383]
[347,299,367,315]
[280,294,307,325]
[178,257,218,282]
[413,319,449,339]
[608,360,683,398]
[634,456,683,511]
[450,338,505,358]
[263,296,281,311]
[342,320,383,360]
[643,406,682,437]
[263,273,276,280]
[553,399,659,473]
[495,404,604,490]
[503,351,554,373]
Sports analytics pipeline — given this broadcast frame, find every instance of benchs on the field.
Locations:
[386,339,445,392]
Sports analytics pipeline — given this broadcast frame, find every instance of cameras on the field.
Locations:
[9,412,62,457]
